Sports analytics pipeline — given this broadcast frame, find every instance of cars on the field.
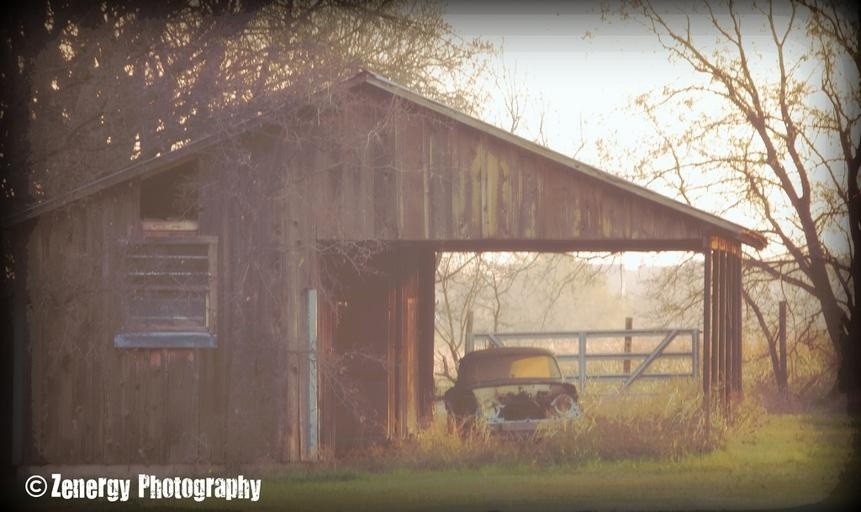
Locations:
[442,346,580,434]
